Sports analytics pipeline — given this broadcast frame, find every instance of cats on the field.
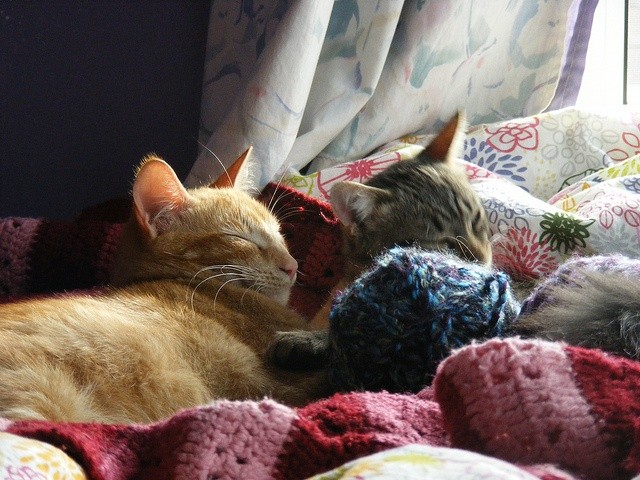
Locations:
[1,143,313,425]
[262,104,640,383]
